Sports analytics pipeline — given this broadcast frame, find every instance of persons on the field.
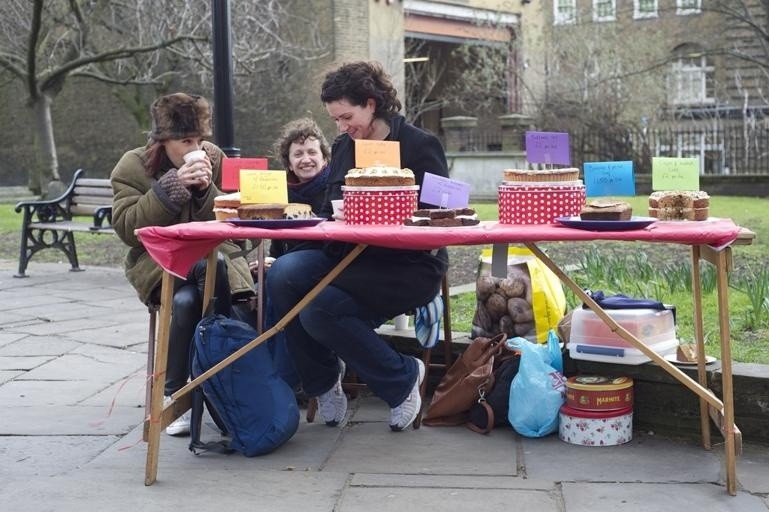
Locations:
[110,92,228,437]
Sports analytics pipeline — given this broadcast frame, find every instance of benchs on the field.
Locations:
[14,168,118,279]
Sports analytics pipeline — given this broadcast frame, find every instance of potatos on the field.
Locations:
[471,270,535,340]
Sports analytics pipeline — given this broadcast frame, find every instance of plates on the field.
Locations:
[220,218,327,230]
[555,214,659,231]
[662,352,717,366]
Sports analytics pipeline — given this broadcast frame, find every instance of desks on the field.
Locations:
[142,218,755,496]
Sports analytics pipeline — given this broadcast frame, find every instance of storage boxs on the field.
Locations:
[568,302,679,365]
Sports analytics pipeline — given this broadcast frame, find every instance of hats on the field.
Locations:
[150,92,213,139]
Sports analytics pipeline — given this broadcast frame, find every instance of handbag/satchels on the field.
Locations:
[421,332,508,434]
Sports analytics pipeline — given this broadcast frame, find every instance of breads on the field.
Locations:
[403,207,480,226]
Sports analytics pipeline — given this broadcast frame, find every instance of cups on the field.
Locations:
[182,149,210,181]
[329,198,346,222]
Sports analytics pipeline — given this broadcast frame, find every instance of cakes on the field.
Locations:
[580,198,633,221]
[677,341,706,361]
[503,168,579,181]
[237,203,312,220]
[647,190,710,220]
[344,167,416,186]
[212,192,240,220]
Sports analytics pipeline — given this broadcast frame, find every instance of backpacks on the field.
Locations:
[186,313,300,458]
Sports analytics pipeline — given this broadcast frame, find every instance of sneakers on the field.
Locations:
[163,395,191,436]
[388,358,425,432]
[318,355,347,427]
